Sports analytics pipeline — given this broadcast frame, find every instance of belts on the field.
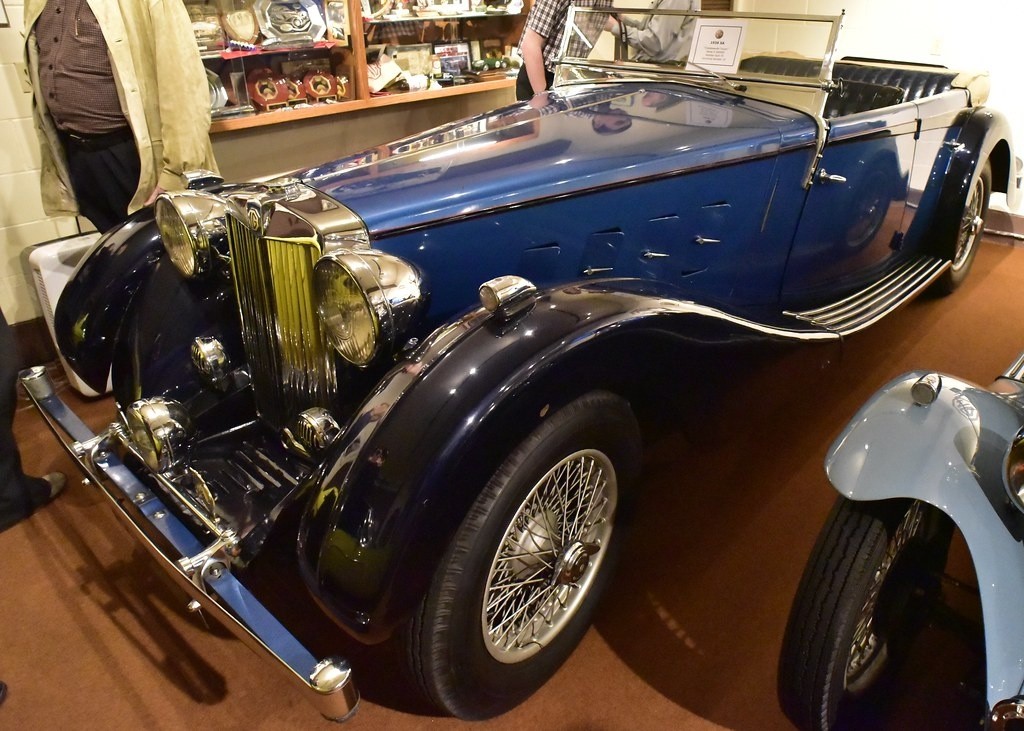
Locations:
[54,124,134,152]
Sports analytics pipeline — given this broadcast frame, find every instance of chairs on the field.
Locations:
[822,79,905,122]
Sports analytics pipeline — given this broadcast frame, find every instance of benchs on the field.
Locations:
[738,57,960,106]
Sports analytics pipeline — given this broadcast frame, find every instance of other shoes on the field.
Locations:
[41,470,66,500]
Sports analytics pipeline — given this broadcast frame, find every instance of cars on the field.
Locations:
[16,3,1017,725]
[777,344,1024,731]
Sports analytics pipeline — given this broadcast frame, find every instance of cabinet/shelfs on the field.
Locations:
[181,0,537,134]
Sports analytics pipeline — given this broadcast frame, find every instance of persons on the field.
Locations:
[515,0,613,99]
[0,307,68,534]
[642,91,668,108]
[486,100,632,136]
[604,0,698,61]
[19,0,223,237]
